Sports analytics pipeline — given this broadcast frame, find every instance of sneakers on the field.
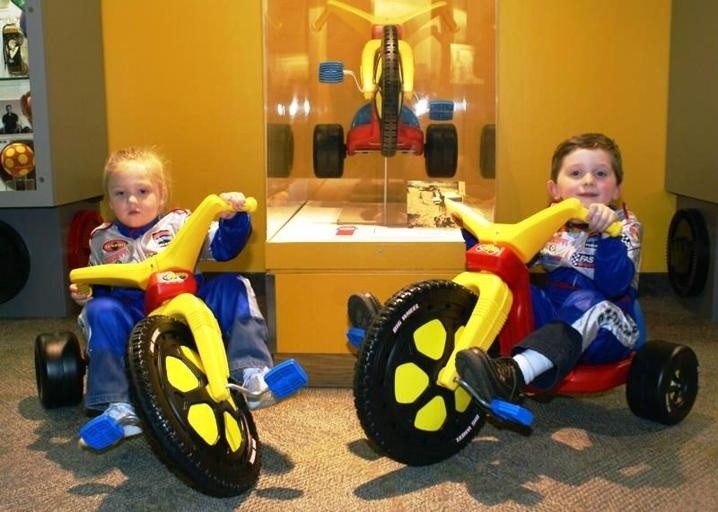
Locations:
[243,363,283,411]
[79,402,142,448]
[344,291,381,326]
[452,345,531,408]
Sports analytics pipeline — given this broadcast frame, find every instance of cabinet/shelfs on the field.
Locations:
[1,0,109,207]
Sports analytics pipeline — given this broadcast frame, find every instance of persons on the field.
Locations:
[2,105,19,133]
[70,147,272,451]
[347,131,642,405]
[7,39,22,71]
